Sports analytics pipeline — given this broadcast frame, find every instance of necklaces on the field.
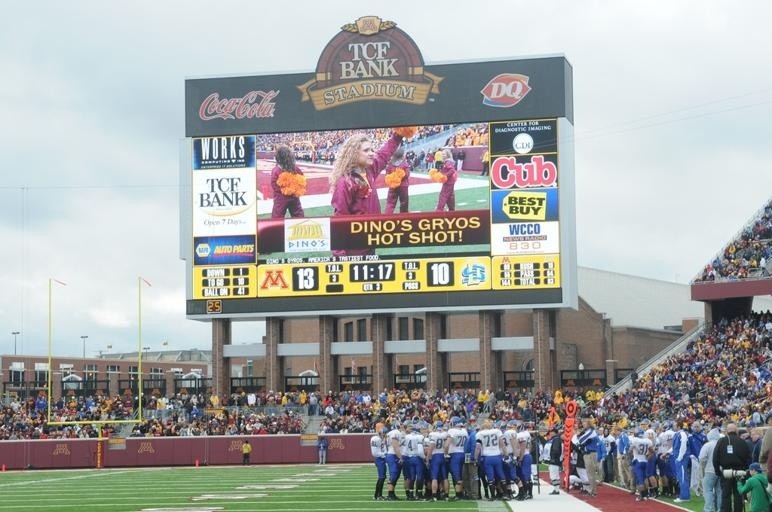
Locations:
[435,148,459,212]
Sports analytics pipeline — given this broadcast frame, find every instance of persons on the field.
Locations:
[270,146,309,218]
[1,389,605,440]
[329,133,405,214]
[242,441,252,466]
[602,311,772,428]
[317,437,328,465]
[255,122,489,176]
[702,200,772,281]
[385,147,412,214]
[372,417,772,512]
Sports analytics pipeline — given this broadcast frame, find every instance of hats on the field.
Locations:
[550,429,557,434]
[750,462,764,471]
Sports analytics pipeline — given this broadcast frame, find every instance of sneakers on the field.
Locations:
[374,495,532,503]
[579,488,597,496]
[619,482,690,502]
[550,490,559,495]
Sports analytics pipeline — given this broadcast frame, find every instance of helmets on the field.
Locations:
[379,415,525,433]
[635,419,673,437]
